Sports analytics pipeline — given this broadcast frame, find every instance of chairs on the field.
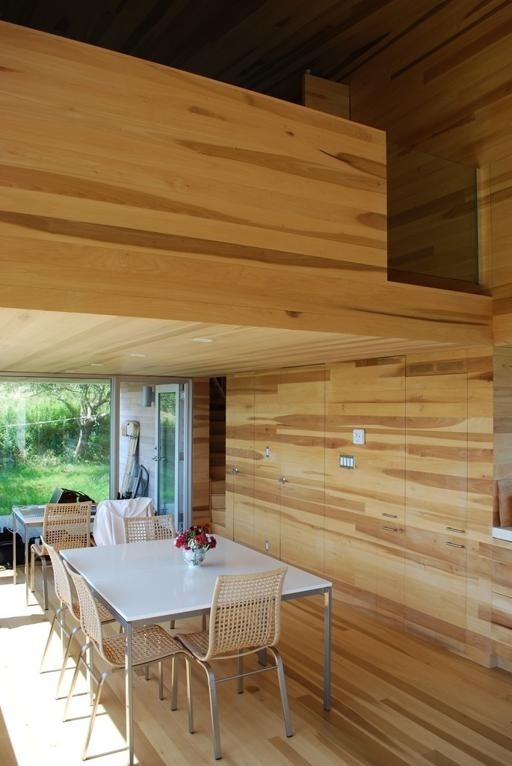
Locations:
[119,513,175,632]
[63,561,197,762]
[31,501,92,611]
[177,522,218,549]
[170,567,295,762]
[42,537,150,719]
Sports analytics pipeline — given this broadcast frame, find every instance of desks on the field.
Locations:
[61,534,333,766]
[12,504,96,607]
